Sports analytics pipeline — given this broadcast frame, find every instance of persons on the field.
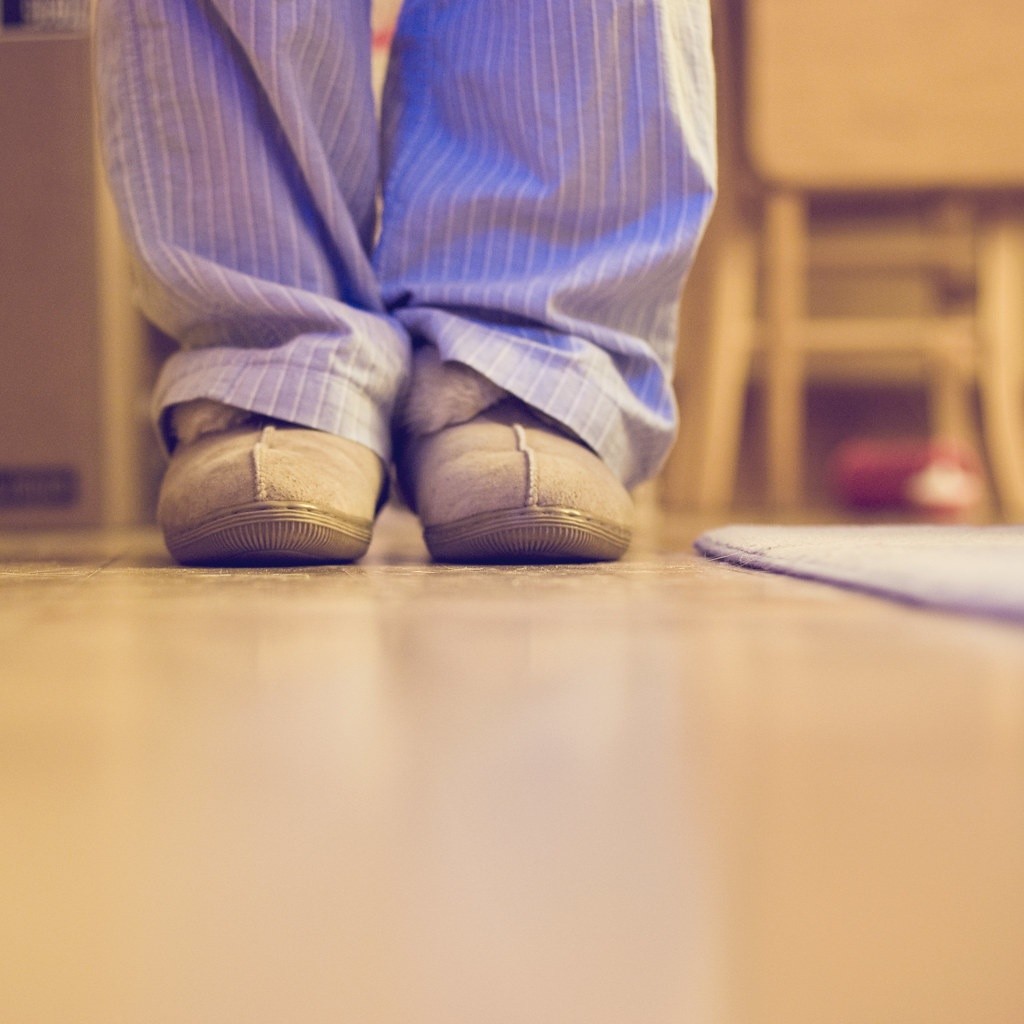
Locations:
[98,0,718,565]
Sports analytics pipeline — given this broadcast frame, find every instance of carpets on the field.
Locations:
[694,521,1024,627]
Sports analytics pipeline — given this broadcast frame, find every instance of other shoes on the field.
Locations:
[157,398,386,565]
[393,345,636,561]
